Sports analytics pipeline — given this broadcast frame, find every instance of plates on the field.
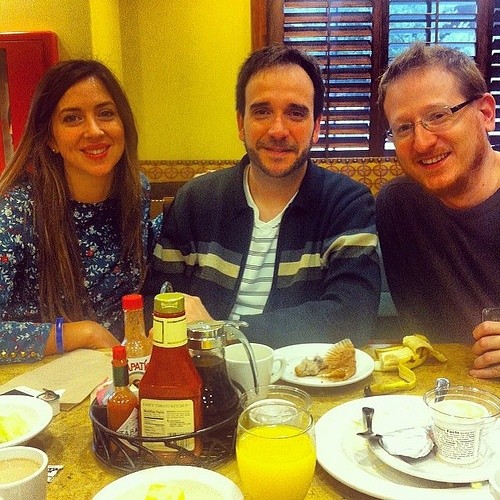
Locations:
[317,394,500,499]
[272,343,377,388]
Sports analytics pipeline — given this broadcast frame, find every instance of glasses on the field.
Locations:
[385,96,479,143]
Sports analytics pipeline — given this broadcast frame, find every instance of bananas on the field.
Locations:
[365,334,447,396]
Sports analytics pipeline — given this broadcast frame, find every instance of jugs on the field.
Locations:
[190,319,259,455]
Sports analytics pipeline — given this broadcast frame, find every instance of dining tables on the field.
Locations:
[0,344,500,500]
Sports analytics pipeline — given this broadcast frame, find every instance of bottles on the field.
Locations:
[141,293,203,465]
[122,293,152,402]
[107,346,141,458]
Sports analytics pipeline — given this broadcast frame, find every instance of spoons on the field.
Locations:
[356,405,384,441]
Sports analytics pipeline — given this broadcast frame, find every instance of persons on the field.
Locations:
[138,43,382,350]
[376,45,500,378]
[0,60,217,364]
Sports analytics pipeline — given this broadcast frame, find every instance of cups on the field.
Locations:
[225,342,286,405]
[38,393,60,417]
[235,385,316,500]
[0,446,48,500]
[481,307,500,323]
[422,387,500,466]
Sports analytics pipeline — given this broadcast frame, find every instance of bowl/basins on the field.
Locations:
[91,465,245,500]
[0,395,53,450]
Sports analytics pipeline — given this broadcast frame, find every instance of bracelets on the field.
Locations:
[56,317,63,353]
